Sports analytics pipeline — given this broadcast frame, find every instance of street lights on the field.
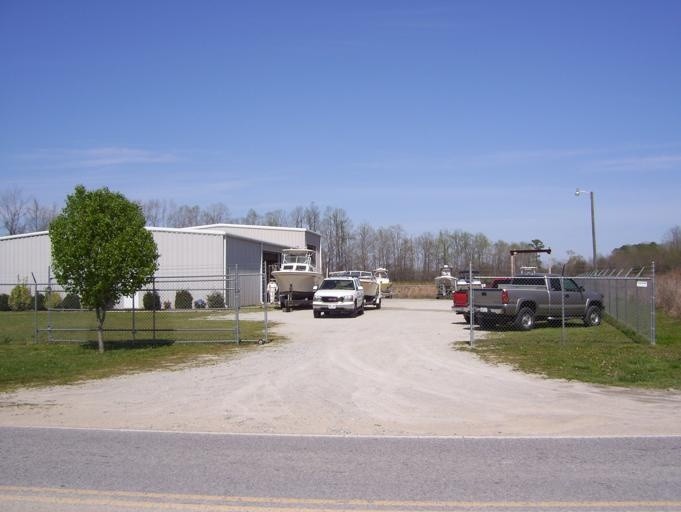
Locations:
[574,190,599,269]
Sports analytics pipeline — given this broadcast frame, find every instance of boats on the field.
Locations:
[373,267,393,293]
[435,265,456,290]
[271,248,322,297]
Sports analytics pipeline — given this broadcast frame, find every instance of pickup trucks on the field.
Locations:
[452,273,604,331]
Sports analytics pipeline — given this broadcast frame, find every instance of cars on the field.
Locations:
[312,277,363,318]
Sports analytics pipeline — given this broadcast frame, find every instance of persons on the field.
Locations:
[266,278,278,305]
